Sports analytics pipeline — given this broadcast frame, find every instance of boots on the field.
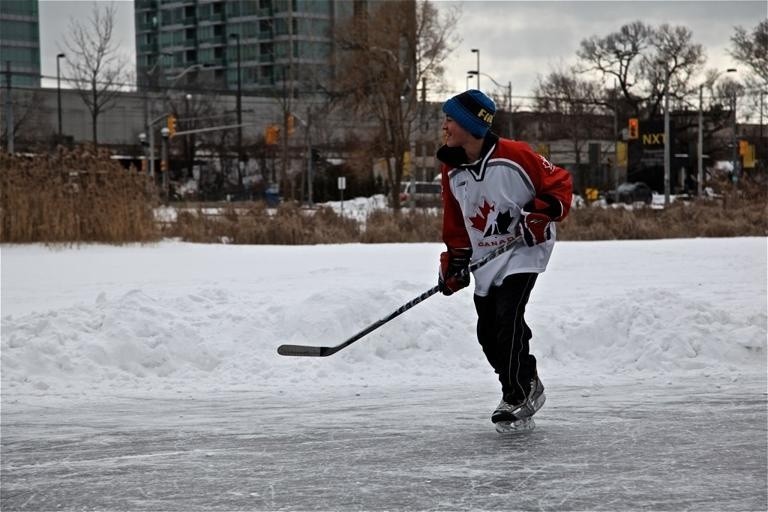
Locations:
[491,397,535,423]
[526,377,546,416]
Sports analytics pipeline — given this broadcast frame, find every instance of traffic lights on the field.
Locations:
[628,119,639,140]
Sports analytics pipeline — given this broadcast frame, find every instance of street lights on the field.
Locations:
[57,53,66,134]
[228,32,243,148]
[468,69,514,140]
[466,75,473,91]
[142,47,217,185]
[696,68,739,196]
[471,49,480,90]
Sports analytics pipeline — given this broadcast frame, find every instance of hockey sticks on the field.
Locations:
[277,235,523,355]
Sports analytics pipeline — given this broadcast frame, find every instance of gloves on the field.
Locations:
[517,205,553,247]
[437,250,473,296]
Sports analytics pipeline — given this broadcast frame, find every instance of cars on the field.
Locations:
[605,182,653,205]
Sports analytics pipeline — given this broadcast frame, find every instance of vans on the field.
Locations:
[387,181,442,207]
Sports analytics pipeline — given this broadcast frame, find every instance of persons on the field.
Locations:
[436,89,574,428]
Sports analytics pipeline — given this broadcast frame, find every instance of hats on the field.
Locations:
[442,90,496,140]
